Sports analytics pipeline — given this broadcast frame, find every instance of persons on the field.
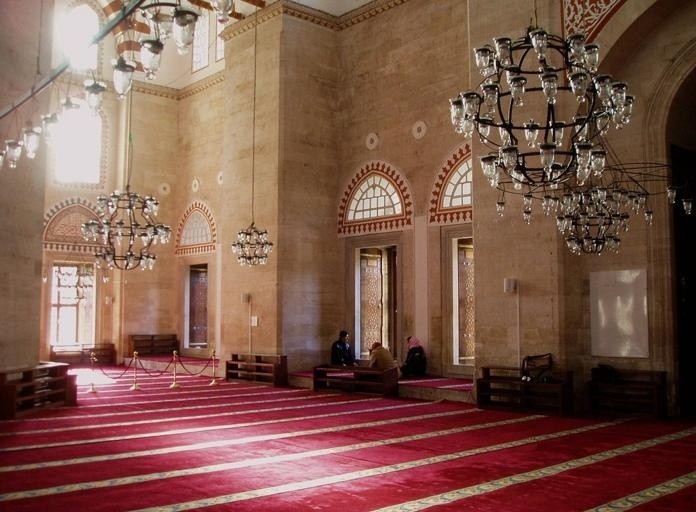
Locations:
[331,330,359,366]
[398,336,427,380]
[367,342,394,369]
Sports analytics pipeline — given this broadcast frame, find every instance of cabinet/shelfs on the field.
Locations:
[313,365,398,393]
[476,365,559,416]
[226,353,287,385]
[128,335,179,357]
[1,363,76,420]
[584,368,667,418]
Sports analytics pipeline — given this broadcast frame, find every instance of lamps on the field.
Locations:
[78,5,171,271]
[496,108,693,257]
[233,4,273,267]
[448,1,633,194]
